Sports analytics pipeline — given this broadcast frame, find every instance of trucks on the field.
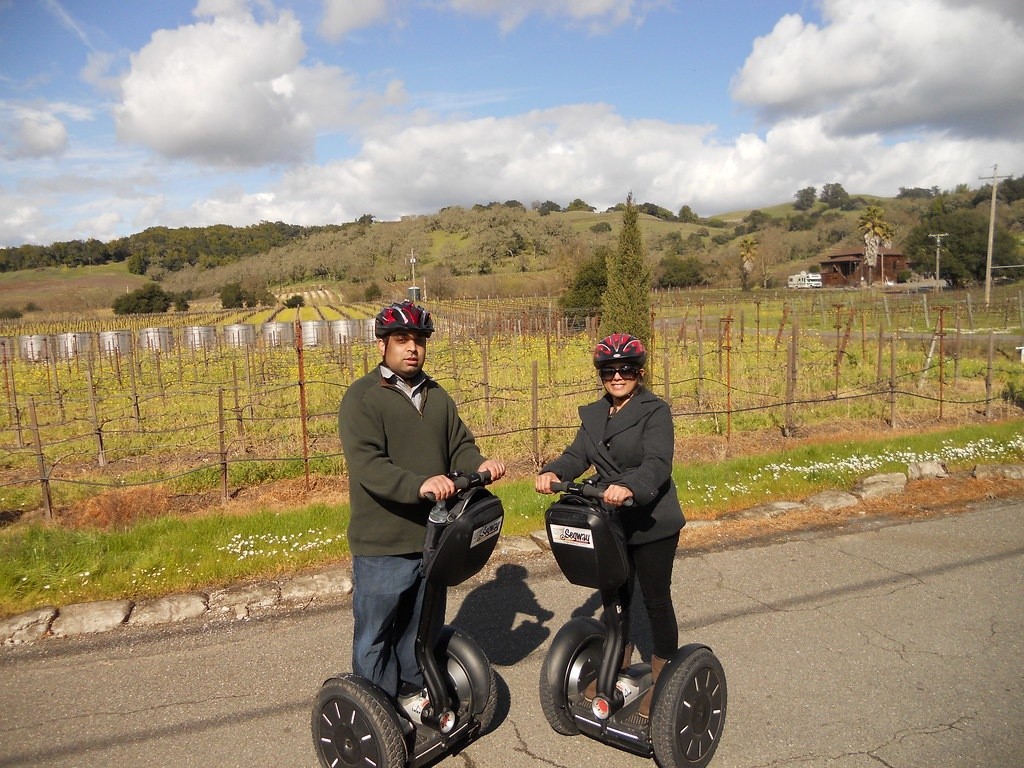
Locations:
[788,275,822,289]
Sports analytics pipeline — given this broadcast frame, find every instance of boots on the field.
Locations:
[637,652,668,718]
[584,642,632,702]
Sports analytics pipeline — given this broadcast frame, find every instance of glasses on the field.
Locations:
[598,367,639,381]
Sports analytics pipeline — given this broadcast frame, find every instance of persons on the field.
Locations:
[534,335,687,719]
[338,297,507,734]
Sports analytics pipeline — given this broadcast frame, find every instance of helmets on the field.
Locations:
[593,333,647,369]
[375,300,435,339]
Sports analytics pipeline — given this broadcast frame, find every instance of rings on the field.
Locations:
[612,497,618,501]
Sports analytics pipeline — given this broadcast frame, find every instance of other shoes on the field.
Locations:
[394,709,413,734]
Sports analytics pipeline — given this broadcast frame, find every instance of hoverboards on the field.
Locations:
[309,468,498,768]
[537,481,728,768]
